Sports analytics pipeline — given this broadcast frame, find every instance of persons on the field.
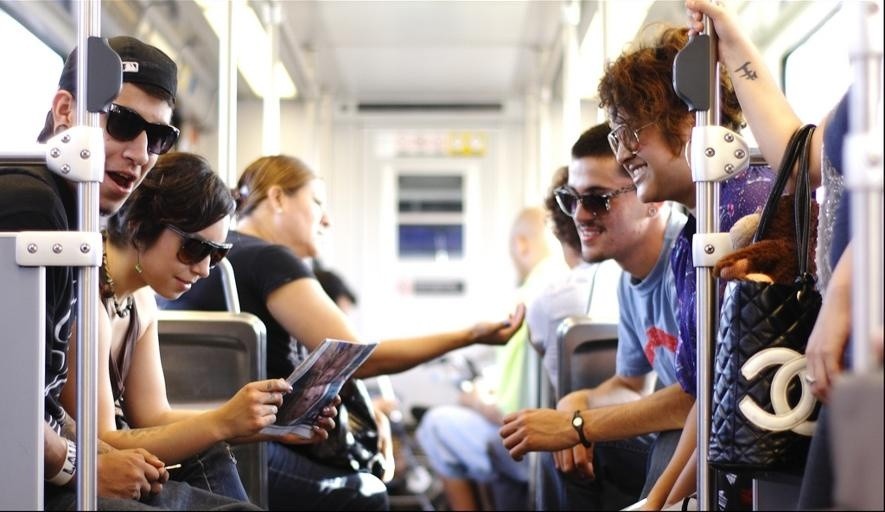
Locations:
[152,152,527,511]
[416,207,571,512]
[285,343,367,425]
[0,37,261,512]
[497,124,688,501]
[291,269,399,447]
[60,153,342,503]
[596,23,820,511]
[525,166,623,403]
[685,0,883,511]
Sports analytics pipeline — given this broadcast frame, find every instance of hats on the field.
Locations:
[54,35,178,99]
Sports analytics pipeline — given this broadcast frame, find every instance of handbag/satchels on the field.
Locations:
[302,373,397,485]
[705,270,825,472]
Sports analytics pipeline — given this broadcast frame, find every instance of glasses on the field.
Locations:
[552,183,637,219]
[163,222,235,269]
[606,123,670,154]
[99,103,181,156]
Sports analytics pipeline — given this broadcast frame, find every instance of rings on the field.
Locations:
[803,372,817,385]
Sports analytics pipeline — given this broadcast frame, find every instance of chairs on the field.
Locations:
[156,254,268,511]
[557,316,622,401]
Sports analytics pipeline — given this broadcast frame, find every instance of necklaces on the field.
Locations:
[102,241,132,319]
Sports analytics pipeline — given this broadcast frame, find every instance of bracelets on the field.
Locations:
[43,437,77,487]
[571,409,594,447]
